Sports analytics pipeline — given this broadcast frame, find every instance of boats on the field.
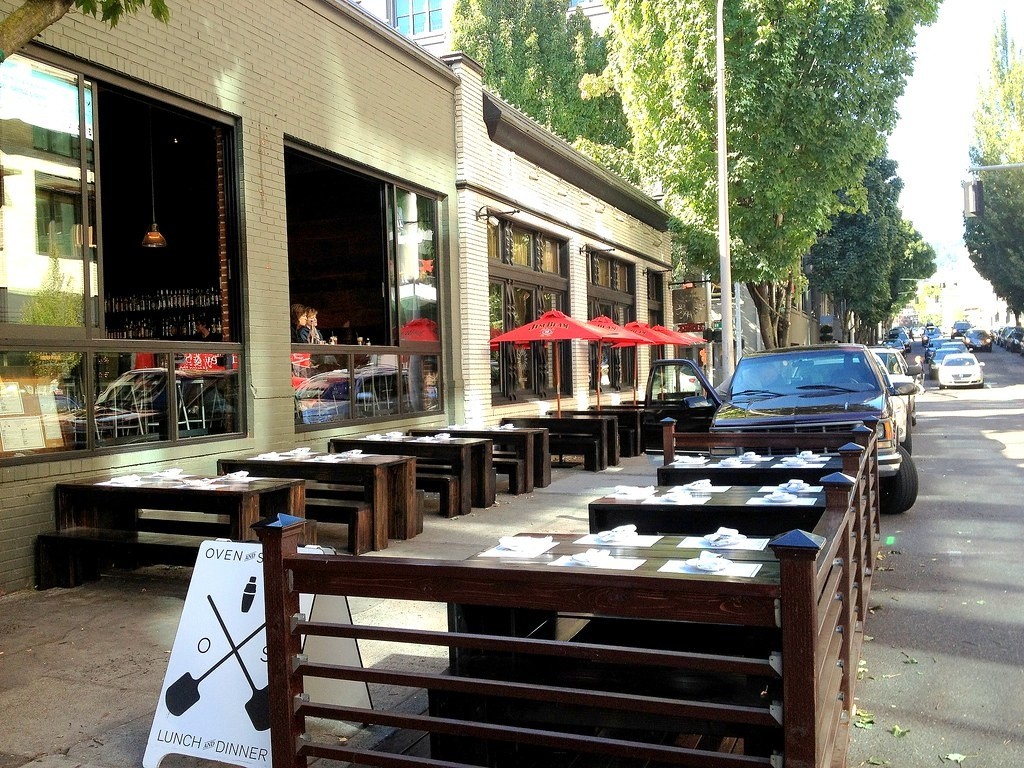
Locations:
[866,317,1024,425]
[786,352,912,458]
[644,342,918,514]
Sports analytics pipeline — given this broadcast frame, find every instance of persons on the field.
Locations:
[291,303,307,343]
[297,304,338,373]
[191,316,218,342]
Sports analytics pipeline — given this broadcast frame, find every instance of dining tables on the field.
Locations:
[32,363,880,768]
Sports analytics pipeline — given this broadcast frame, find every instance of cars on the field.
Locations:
[293,363,414,425]
[54,366,238,448]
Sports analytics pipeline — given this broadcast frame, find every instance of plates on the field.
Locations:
[447,421,514,431]
[673,450,823,469]
[258,446,362,462]
[111,467,250,486]
[365,431,451,443]
[498,524,746,572]
[609,476,810,503]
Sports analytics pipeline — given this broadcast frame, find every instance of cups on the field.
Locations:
[318,335,371,346]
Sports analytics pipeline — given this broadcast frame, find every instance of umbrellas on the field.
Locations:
[579,313,657,411]
[652,323,692,401]
[611,320,691,406]
[487,309,619,469]
[674,332,708,392]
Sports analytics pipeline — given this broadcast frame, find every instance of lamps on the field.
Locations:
[141,104,168,248]
[476,205,500,227]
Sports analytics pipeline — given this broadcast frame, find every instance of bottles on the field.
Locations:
[102,286,222,341]
